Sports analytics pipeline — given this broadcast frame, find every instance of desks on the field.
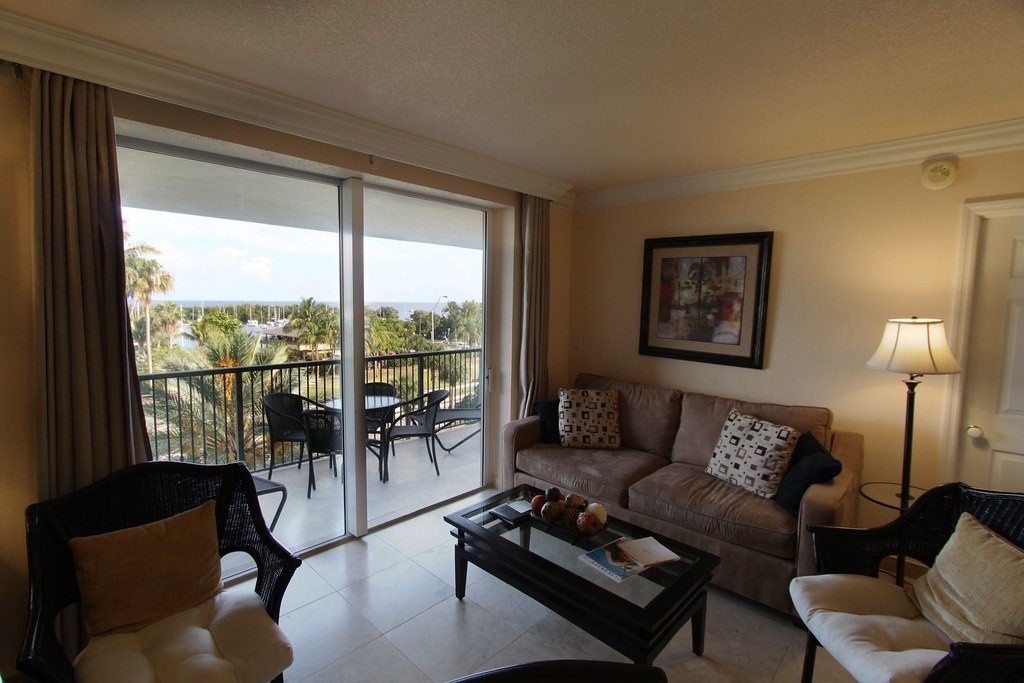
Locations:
[443,483,722,666]
[325,396,402,482]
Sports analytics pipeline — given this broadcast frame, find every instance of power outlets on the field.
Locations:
[574,336,582,349]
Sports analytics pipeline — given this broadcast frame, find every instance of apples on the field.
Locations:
[531,495,545,512]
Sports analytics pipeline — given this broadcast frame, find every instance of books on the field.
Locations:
[489,495,531,528]
[577,535,681,584]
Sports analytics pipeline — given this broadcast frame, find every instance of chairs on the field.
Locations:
[302,410,341,499]
[386,390,450,481]
[789,480,1024,683]
[15,461,302,683]
[262,393,337,490]
[410,403,482,454]
[366,383,397,456]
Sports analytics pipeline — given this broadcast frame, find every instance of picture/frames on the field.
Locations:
[638,230,775,371]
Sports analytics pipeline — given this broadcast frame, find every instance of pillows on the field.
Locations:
[776,431,842,517]
[558,388,621,449]
[705,408,801,499]
[903,512,1024,643]
[69,500,224,635]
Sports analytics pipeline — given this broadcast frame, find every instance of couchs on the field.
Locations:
[504,374,865,630]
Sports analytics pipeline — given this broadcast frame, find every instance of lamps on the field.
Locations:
[860,316,964,588]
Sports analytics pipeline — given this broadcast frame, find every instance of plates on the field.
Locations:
[530,511,610,538]
